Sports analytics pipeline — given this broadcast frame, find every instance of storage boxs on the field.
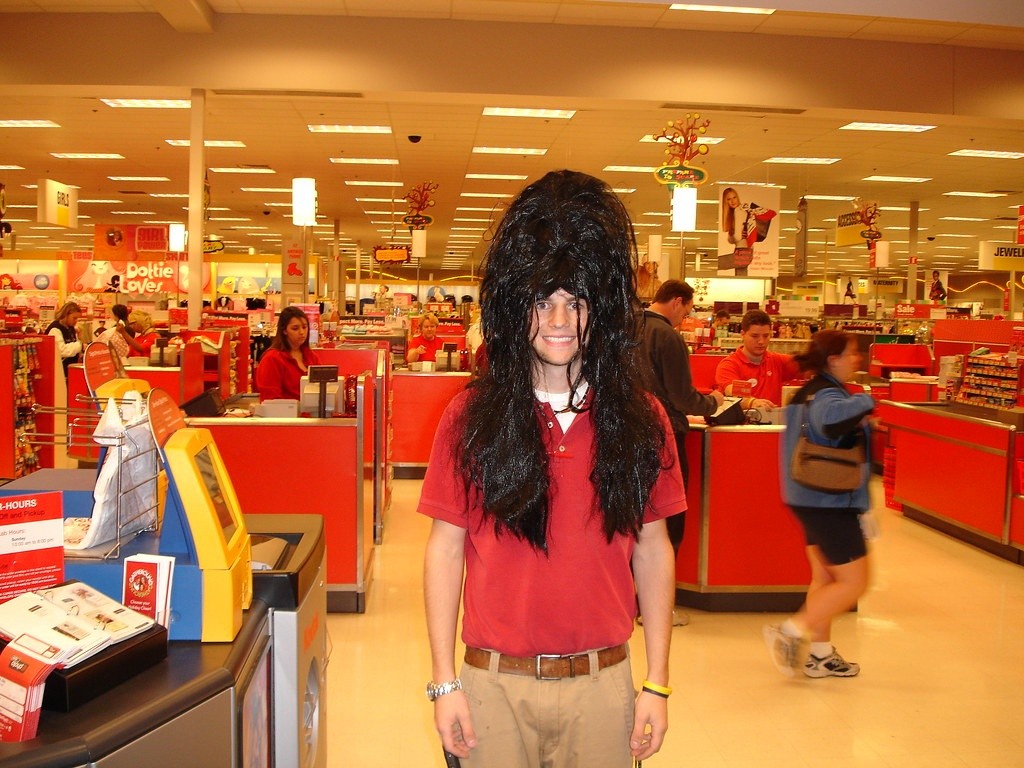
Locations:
[824,304,868,317]
[714,300,759,315]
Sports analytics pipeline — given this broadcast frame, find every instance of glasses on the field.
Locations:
[126,320,135,327]
[683,305,690,319]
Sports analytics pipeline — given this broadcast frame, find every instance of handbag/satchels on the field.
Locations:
[746,215,758,247]
[749,202,777,223]
[790,384,868,491]
[852,293,856,298]
[741,203,771,242]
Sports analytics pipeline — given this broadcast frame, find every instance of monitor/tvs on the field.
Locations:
[299,376,344,412]
[150,344,177,365]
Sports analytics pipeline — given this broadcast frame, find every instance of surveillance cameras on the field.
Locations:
[408,135,421,143]
[927,237,935,240]
[263,211,270,215]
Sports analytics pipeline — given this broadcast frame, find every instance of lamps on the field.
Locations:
[169,224,185,306]
[291,178,317,301]
[672,187,697,280]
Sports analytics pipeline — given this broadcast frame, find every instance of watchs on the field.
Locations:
[427,679,461,701]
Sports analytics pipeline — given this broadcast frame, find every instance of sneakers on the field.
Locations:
[802,645,860,678]
[761,623,802,677]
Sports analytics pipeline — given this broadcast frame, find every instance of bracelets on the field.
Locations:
[642,681,670,697]
[748,398,756,409]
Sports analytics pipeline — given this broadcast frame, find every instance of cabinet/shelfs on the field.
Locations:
[0,275,1024,768]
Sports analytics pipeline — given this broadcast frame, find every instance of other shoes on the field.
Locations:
[636,605,689,627]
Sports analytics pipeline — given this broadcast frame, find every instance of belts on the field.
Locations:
[463,644,627,680]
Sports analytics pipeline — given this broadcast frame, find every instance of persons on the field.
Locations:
[763,328,875,678]
[929,270,947,302]
[715,310,816,424]
[710,312,730,344]
[719,188,754,276]
[374,285,390,308]
[110,304,163,367]
[632,279,724,626]
[405,313,446,371]
[417,169,685,768]
[44,301,89,384]
[255,306,323,418]
